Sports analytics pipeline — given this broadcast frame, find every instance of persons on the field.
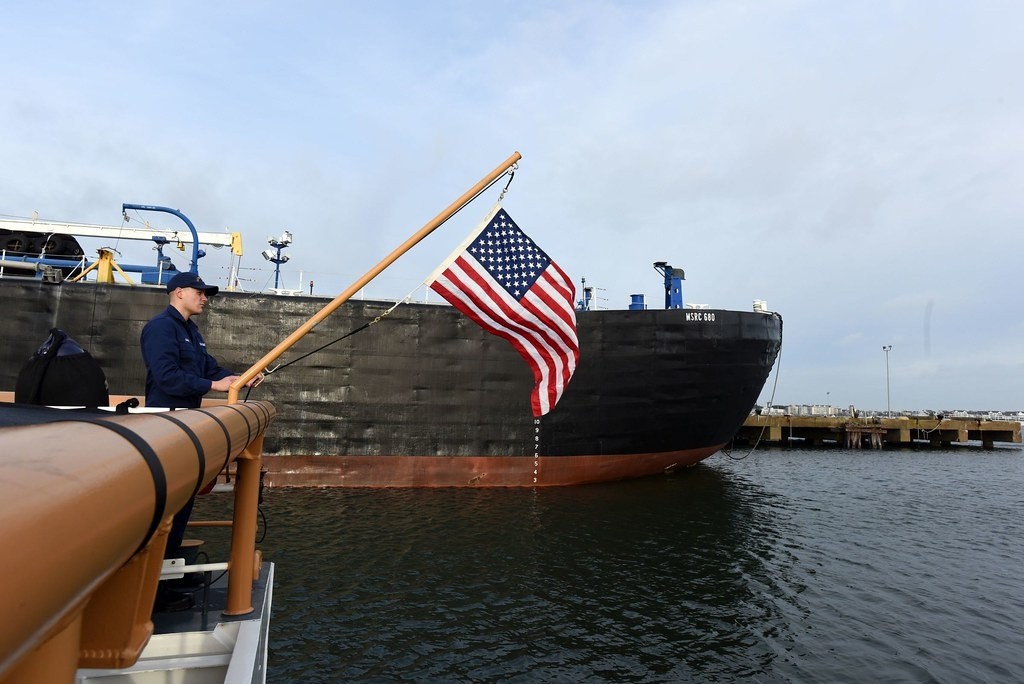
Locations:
[139,273,264,559]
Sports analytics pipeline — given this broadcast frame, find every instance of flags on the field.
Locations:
[424,202,580,416]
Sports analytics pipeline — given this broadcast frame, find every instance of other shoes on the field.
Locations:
[160,593,193,611]
[184,574,204,588]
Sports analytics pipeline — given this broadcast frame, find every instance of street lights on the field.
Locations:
[882,345,892,418]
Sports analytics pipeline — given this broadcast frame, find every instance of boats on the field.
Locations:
[0,214,786,488]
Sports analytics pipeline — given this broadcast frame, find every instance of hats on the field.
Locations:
[167,272,219,297]
